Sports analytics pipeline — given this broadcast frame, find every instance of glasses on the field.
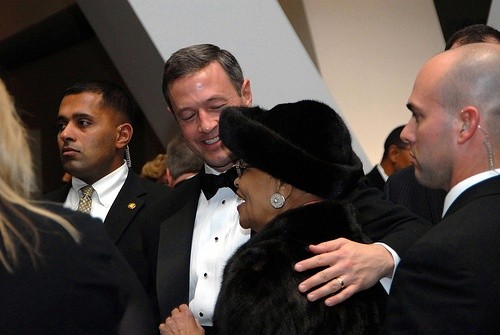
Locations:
[235,159,250,178]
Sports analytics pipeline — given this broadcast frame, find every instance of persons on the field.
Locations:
[0,22,499,335]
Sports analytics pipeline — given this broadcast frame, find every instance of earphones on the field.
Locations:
[462,123,469,130]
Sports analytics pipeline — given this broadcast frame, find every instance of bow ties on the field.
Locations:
[198,166,245,200]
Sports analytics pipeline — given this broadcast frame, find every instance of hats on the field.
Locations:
[219,100,363,202]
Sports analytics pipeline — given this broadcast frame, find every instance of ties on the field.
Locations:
[77,185,96,215]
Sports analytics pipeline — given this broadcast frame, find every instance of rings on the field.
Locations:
[337,277,346,289]
